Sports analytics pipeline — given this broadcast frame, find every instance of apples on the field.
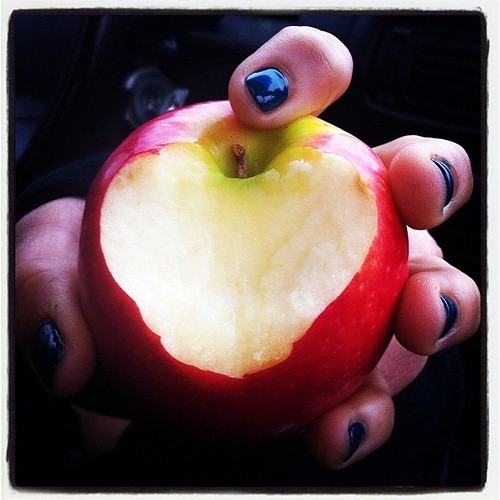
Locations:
[76,98,410,445]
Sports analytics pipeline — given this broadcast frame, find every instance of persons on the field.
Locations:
[10,24,482,487]
